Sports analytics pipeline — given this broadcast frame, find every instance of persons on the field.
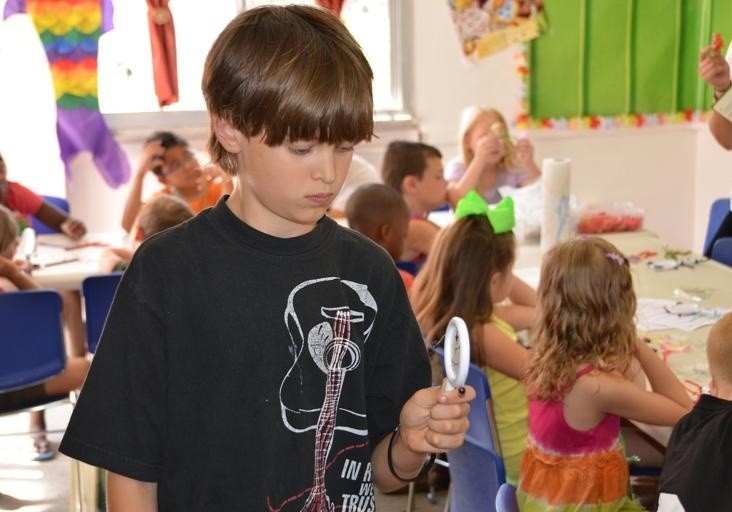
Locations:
[0,127,232,456]
[346,105,732,511]
[698,31,732,149]
[56,4,478,511]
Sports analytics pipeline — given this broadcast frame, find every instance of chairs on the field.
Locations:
[82,274,130,350]
[30,194,74,236]
[407,257,673,512]
[1,290,85,512]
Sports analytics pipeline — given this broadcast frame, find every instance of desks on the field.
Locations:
[427,194,730,458]
[1,232,145,294]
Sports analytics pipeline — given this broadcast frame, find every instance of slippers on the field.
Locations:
[32,437,53,460]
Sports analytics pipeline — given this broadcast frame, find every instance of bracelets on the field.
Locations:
[388,423,436,483]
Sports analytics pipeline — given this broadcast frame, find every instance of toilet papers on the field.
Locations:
[542,158,573,211]
